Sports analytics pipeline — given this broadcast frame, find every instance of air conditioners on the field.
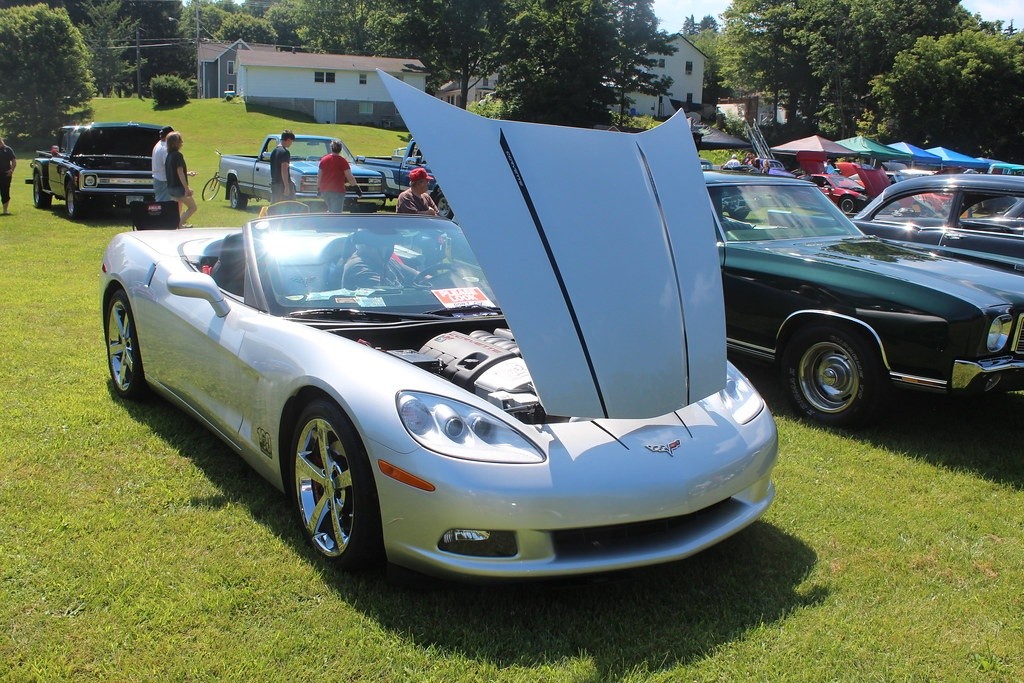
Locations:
[686,71,692,75]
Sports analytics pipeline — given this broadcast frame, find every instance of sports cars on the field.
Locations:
[104,66,780,582]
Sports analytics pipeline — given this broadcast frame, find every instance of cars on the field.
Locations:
[697,168,1024,427]
[754,156,1024,278]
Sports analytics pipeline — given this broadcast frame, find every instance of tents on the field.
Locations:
[834,131,912,161]
[977,157,1005,166]
[886,141,943,168]
[924,146,990,170]
[689,114,755,151]
[770,133,860,157]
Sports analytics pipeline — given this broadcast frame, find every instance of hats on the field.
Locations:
[409,168,433,180]
[732,154,737,158]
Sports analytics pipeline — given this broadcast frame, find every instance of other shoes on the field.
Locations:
[178,223,193,228]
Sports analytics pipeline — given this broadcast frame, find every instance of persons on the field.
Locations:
[797,169,813,180]
[268,129,298,204]
[150,125,199,228]
[825,159,835,174]
[47,144,62,157]
[0,137,16,215]
[396,167,458,267]
[340,228,432,291]
[724,155,742,170]
[741,151,770,174]
[318,140,366,213]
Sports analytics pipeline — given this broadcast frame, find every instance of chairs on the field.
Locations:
[314,231,357,291]
[210,231,284,298]
[129,200,180,231]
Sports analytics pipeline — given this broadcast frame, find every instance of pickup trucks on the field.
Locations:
[24,122,167,220]
[218,133,385,210]
[355,138,455,220]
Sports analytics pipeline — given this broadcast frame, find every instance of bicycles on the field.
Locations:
[200,148,223,202]
